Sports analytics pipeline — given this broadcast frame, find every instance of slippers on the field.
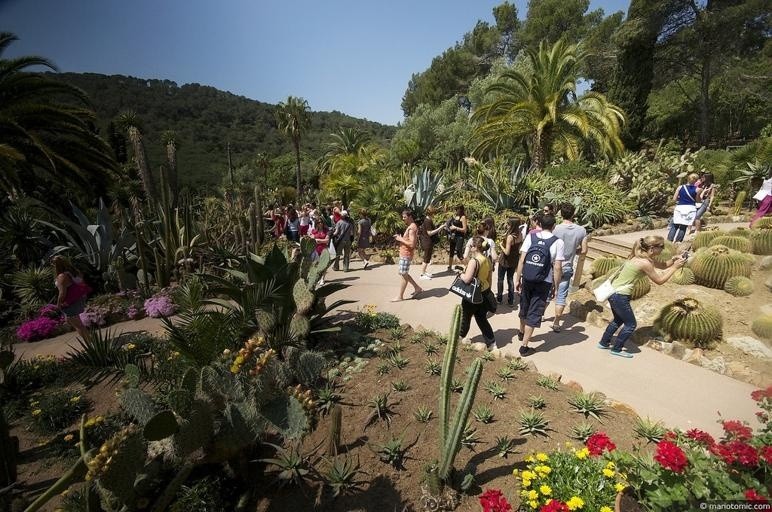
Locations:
[411,291,425,299]
[390,296,403,302]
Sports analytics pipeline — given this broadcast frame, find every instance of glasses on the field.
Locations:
[648,243,666,250]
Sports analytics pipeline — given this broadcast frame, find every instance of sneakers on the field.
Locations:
[596,342,613,349]
[610,349,633,358]
[419,271,433,280]
[446,266,451,273]
[518,332,524,341]
[552,322,561,332]
[486,340,498,351]
[332,261,372,272]
[496,297,515,307]
[519,346,536,357]
[541,315,545,322]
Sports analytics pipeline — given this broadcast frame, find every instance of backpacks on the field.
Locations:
[522,233,559,284]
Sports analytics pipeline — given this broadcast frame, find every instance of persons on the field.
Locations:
[667,173,704,243]
[53,261,81,314]
[687,173,717,235]
[596,235,688,358]
[265,201,587,356]
[749,166,772,228]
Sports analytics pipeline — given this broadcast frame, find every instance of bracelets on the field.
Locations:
[671,257,678,262]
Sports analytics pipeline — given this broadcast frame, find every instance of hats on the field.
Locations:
[339,209,350,217]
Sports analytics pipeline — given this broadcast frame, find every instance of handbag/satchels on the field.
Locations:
[332,236,341,250]
[448,257,483,304]
[750,186,769,202]
[592,279,616,303]
[368,234,376,245]
[283,220,289,235]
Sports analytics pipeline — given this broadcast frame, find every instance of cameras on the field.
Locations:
[682,251,689,259]
